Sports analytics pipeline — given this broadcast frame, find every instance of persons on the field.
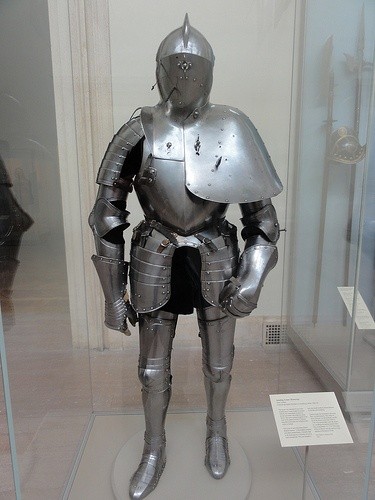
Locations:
[88,12,283,499]
